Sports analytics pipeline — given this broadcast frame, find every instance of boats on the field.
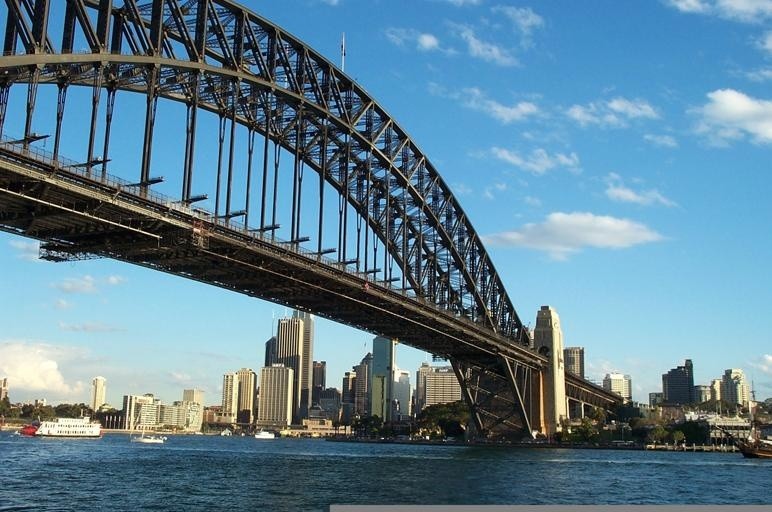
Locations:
[713,421,772,458]
[32,416,104,439]
[255,431,275,439]
[19,423,38,435]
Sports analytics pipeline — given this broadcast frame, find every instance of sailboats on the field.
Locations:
[129,395,164,444]
